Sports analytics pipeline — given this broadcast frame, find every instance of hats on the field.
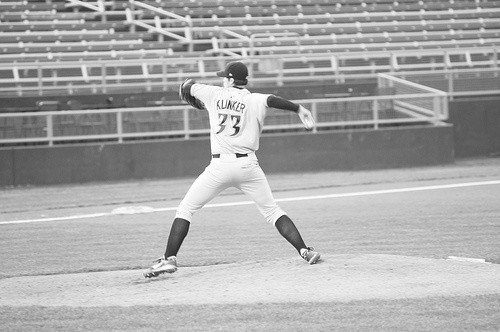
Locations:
[216,61,248,80]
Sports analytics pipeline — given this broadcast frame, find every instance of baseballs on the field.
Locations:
[302,116,314,129]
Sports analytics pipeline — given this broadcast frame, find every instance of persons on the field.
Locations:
[143,61,320,279]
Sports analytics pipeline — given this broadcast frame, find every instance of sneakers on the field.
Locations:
[142,254,177,278]
[301,247,320,265]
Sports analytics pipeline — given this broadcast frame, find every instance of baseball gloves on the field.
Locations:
[184,96,206,110]
